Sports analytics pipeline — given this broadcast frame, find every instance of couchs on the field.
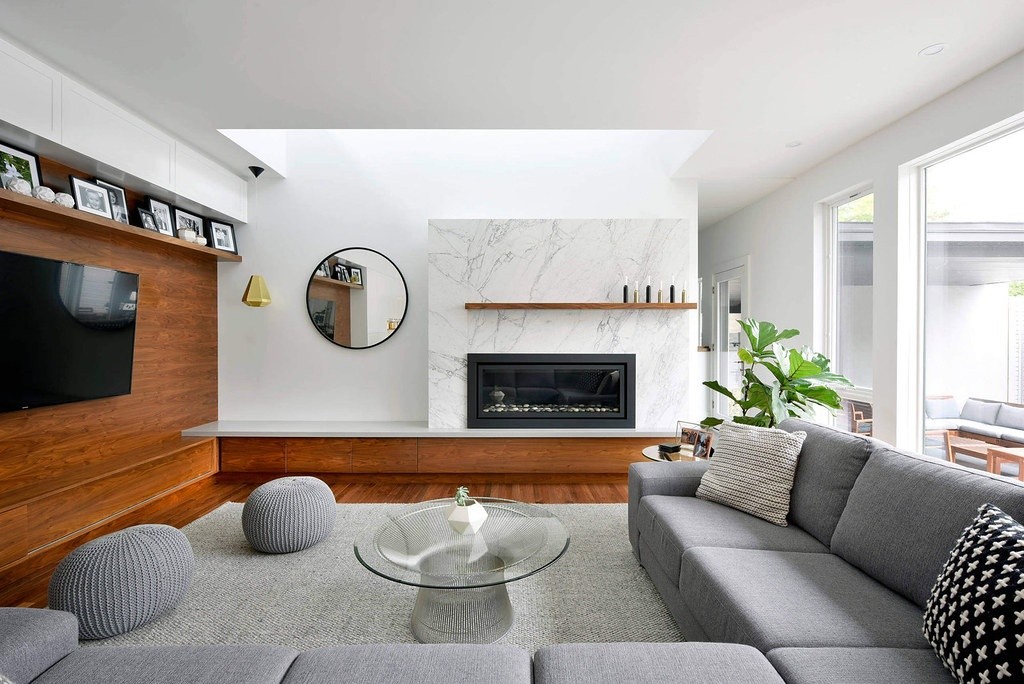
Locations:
[628,416,1024,684]
[0,606,788,684]
[483,369,620,407]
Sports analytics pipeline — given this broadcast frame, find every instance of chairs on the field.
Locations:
[922,429,952,463]
[849,402,872,437]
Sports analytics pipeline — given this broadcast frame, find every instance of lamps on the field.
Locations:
[385,318,399,335]
[240,274,273,309]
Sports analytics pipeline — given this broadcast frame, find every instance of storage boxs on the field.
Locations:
[659,441,682,454]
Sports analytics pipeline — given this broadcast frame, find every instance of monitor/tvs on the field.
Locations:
[0,249,140,414]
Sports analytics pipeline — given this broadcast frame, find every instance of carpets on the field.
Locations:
[77,499,686,647]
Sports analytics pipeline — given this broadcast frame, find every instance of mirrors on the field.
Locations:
[305,246,409,350]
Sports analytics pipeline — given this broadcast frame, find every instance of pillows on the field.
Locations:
[921,502,1024,684]
[690,420,807,529]
[595,370,620,396]
[574,368,610,395]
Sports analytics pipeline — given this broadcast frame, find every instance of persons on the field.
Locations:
[695,436,710,457]
[216,229,222,244]
[85,189,104,212]
[690,433,697,442]
[320,264,326,276]
[109,189,120,219]
[142,213,155,228]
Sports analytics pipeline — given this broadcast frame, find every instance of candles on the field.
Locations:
[635,281,638,290]
[671,274,675,284]
[659,281,663,290]
[683,279,686,290]
[647,274,650,285]
[624,276,628,285]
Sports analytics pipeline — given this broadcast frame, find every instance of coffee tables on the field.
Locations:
[355,494,572,645]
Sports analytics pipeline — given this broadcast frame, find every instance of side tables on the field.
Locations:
[642,443,712,462]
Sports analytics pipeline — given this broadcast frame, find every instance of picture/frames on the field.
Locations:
[314,259,331,278]
[315,312,326,326]
[144,194,176,237]
[337,263,347,272]
[350,267,363,285]
[693,430,714,460]
[206,218,238,255]
[343,269,351,283]
[333,265,341,279]
[68,173,115,220]
[134,207,160,234]
[172,205,206,239]
[336,271,342,281]
[674,420,710,452]
[0,139,44,190]
[92,176,132,225]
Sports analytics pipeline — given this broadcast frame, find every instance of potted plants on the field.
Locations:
[488,384,505,402]
[447,486,488,536]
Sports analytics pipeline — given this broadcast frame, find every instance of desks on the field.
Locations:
[987,446,1024,482]
[949,443,1015,476]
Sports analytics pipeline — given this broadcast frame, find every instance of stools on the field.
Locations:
[45,523,195,639]
[243,473,338,556]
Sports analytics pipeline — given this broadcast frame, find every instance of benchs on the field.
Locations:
[960,397,1024,449]
[919,394,961,437]
